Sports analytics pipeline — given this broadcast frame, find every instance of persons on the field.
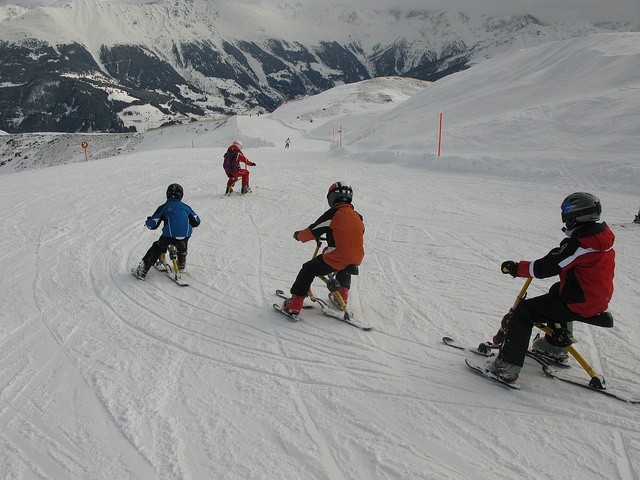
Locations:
[483,191,616,381]
[284,138,291,148]
[131,183,201,280]
[283,180,366,318]
[222,141,256,195]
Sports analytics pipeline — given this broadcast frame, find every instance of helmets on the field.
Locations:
[327,181,354,207]
[166,183,183,200]
[560,192,601,230]
[233,140,243,150]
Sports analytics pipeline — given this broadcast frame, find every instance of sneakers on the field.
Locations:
[225,186,233,194]
[241,186,251,194]
[485,358,523,384]
[176,251,188,270]
[136,262,150,278]
[328,286,349,311]
[282,294,305,318]
[532,337,571,361]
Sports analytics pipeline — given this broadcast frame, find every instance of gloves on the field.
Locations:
[293,230,299,241]
[252,163,256,166]
[501,261,519,279]
